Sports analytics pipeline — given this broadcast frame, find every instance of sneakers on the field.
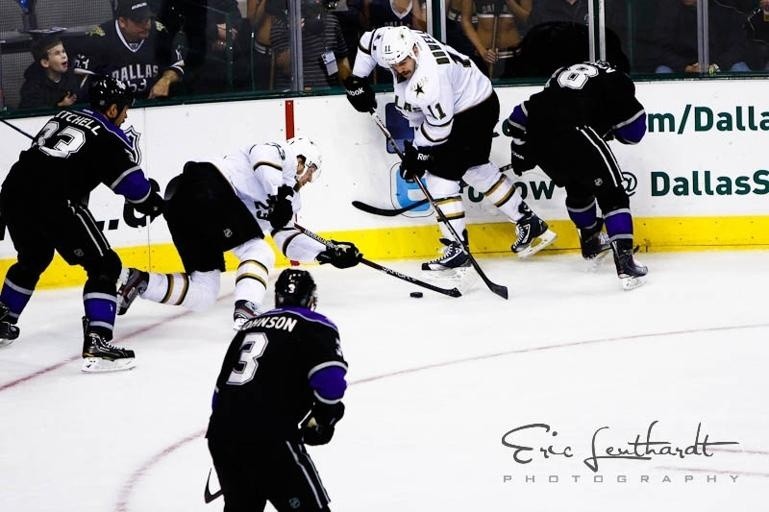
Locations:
[117,268,148,315]
[235,300,261,322]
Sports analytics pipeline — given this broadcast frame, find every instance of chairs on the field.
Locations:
[0,0,116,110]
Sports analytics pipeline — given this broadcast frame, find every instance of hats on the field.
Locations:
[119,0,156,20]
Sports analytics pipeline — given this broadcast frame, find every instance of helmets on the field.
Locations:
[89,75,131,99]
[382,26,414,64]
[275,269,316,311]
[288,137,320,169]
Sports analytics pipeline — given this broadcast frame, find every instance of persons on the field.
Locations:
[0,86,165,376]
[0,1,767,119]
[203,268,350,512]
[114,134,362,321]
[502,58,651,280]
[344,24,549,272]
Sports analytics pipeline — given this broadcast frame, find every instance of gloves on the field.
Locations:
[134,191,164,216]
[316,240,362,268]
[269,195,292,228]
[400,139,432,182]
[305,403,344,446]
[512,140,534,175]
[344,76,376,111]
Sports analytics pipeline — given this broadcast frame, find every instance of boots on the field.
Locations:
[83,316,135,363]
[511,201,548,252]
[577,219,610,252]
[0,302,19,340]
[422,229,471,269]
[610,240,648,278]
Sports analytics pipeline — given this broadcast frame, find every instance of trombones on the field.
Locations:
[368,106,508,300]
